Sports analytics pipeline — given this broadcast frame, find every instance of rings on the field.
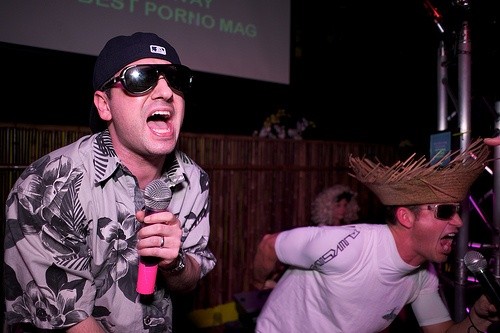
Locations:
[160,236,164,248]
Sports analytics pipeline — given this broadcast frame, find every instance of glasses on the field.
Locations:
[100,64,197,97]
[408,203,463,220]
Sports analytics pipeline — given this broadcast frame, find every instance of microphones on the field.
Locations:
[135,179,172,295]
[464,250,500,318]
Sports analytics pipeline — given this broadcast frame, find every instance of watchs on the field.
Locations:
[159,247,187,277]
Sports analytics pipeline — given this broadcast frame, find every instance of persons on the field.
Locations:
[250,135,500,333]
[0,32,218,333]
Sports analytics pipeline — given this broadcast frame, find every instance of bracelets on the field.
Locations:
[468,315,485,333]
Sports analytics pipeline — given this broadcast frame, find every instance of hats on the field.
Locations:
[88,32,182,134]
[348,137,500,206]
